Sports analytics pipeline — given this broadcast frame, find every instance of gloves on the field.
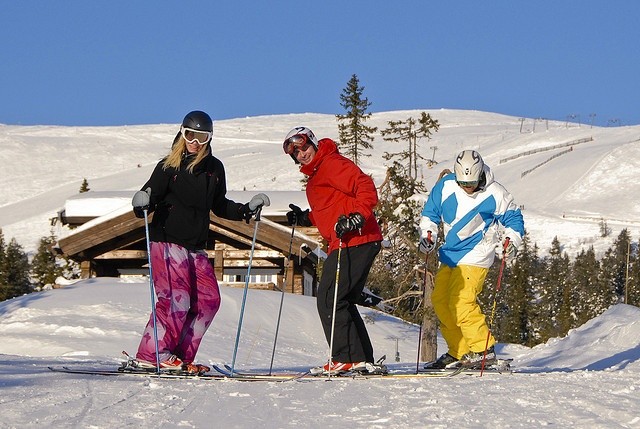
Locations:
[286,203,312,228]
[243,194,270,224]
[132,187,152,208]
[502,241,517,263]
[419,238,434,255]
[334,213,364,239]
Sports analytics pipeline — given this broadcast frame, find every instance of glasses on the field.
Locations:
[282,134,309,154]
[178,126,213,144]
[458,180,481,189]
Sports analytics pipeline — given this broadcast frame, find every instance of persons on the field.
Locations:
[419,150,525,370]
[283,127,383,376]
[132,110,270,369]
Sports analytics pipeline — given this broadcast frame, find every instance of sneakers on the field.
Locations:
[134,353,184,371]
[183,362,210,374]
[310,359,368,374]
[423,352,459,369]
[445,345,498,371]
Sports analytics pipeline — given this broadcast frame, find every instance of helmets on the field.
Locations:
[455,150,484,183]
[181,110,215,134]
[283,126,318,149]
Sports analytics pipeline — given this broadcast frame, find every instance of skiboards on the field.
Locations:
[412,366,516,374]
[212,362,469,380]
[48,366,311,382]
